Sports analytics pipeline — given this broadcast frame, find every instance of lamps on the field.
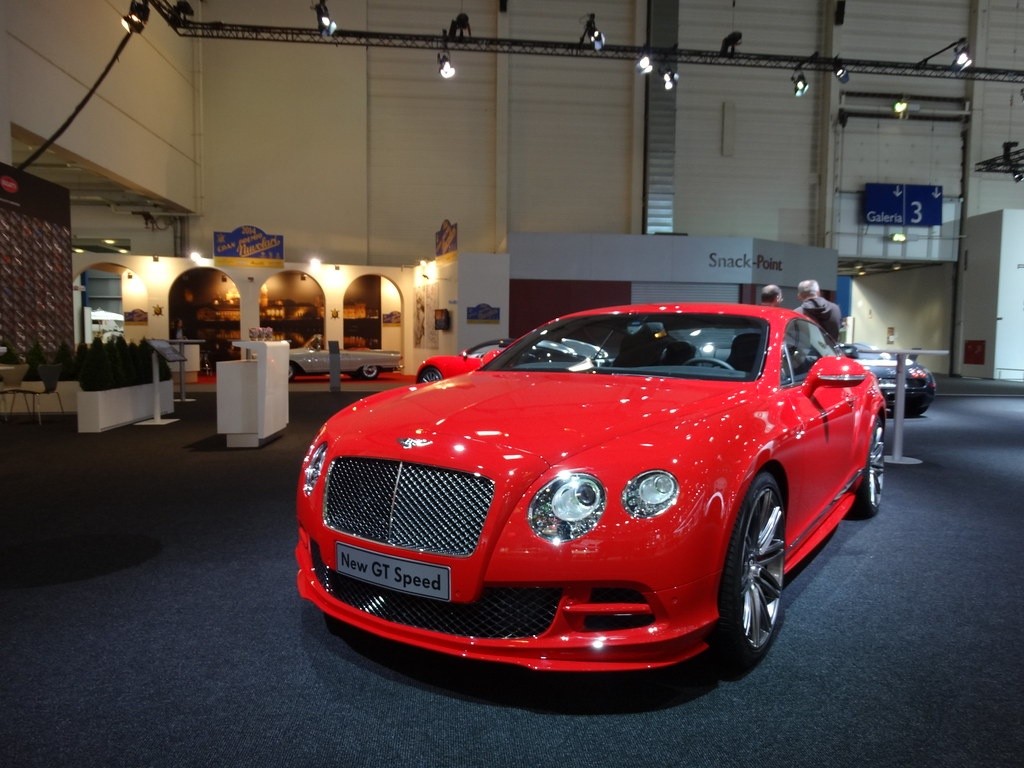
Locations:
[658,66,680,90]
[916,37,973,72]
[889,233,908,242]
[831,54,848,84]
[436,29,456,78]
[121,1,149,34]
[634,45,654,74]
[316,0,337,36]
[791,67,809,97]
[580,13,605,51]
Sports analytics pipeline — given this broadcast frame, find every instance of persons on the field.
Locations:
[760,285,783,307]
[792,280,841,341]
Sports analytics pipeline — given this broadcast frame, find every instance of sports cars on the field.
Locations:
[415,338,516,383]
[288,334,404,382]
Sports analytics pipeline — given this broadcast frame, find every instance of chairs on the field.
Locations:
[612,332,677,368]
[0,362,31,422]
[721,332,761,372]
[8,363,64,424]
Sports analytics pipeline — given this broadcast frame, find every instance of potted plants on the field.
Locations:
[0,338,174,434]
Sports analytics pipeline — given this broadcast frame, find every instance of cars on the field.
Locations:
[836,343,936,417]
[294,301,887,673]
